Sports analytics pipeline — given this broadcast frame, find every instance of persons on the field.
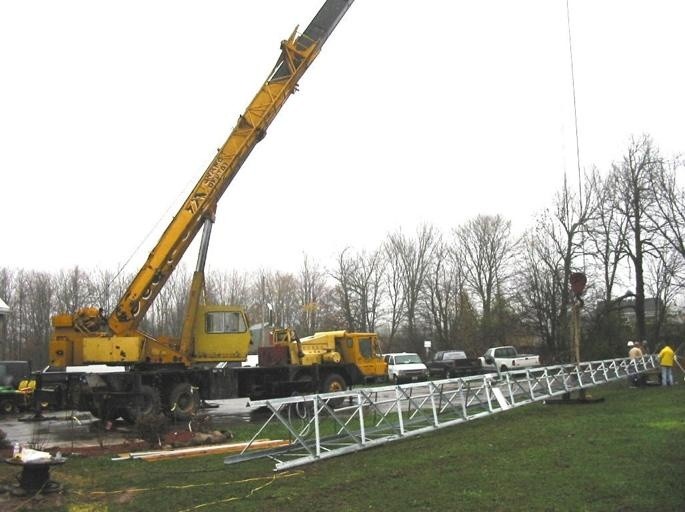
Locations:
[629,340,649,386]
[657,342,674,385]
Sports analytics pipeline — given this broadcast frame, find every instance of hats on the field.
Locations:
[628,341,634,346]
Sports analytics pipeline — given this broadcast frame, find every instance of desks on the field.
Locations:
[5,458,66,490]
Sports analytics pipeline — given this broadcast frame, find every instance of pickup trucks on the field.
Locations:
[376,345,541,385]
